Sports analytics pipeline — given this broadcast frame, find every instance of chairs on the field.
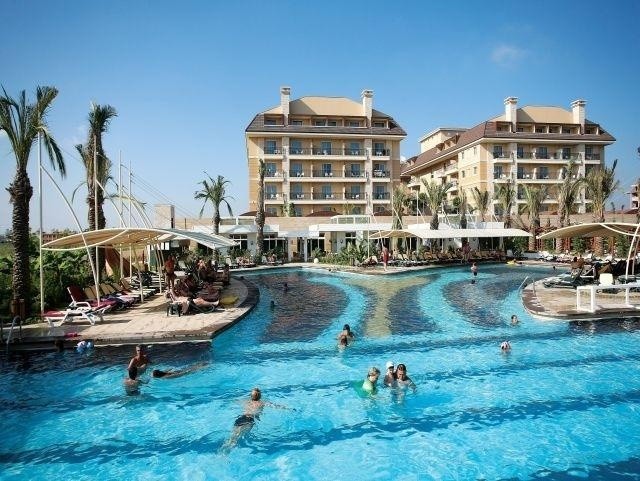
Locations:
[40,271,165,327]
[361,248,527,266]
[165,261,231,317]
[535,253,639,290]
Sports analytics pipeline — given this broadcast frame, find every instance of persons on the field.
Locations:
[382,245,390,271]
[392,388,406,406]
[151,363,209,379]
[568,251,600,280]
[471,261,478,274]
[123,365,145,392]
[339,324,354,339]
[282,281,289,291]
[163,246,230,311]
[384,361,396,390]
[510,314,518,325]
[395,364,416,393]
[362,368,381,395]
[337,335,349,352]
[126,344,151,371]
[270,300,277,309]
[221,389,286,454]
[462,242,472,262]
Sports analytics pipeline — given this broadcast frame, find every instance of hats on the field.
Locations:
[386,362,394,369]
[500,341,511,349]
[367,367,380,377]
